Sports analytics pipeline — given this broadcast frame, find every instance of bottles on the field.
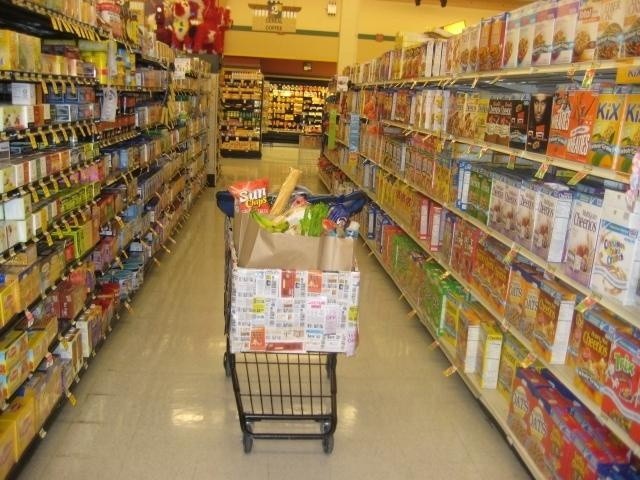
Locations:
[344,221,361,238]
[26,0,140,47]
[79,38,210,259]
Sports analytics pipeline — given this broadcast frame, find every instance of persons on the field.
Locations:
[530,94,550,133]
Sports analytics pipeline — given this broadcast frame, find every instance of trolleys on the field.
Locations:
[218,184,339,456]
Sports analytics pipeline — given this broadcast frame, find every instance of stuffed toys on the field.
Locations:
[164,0,234,56]
